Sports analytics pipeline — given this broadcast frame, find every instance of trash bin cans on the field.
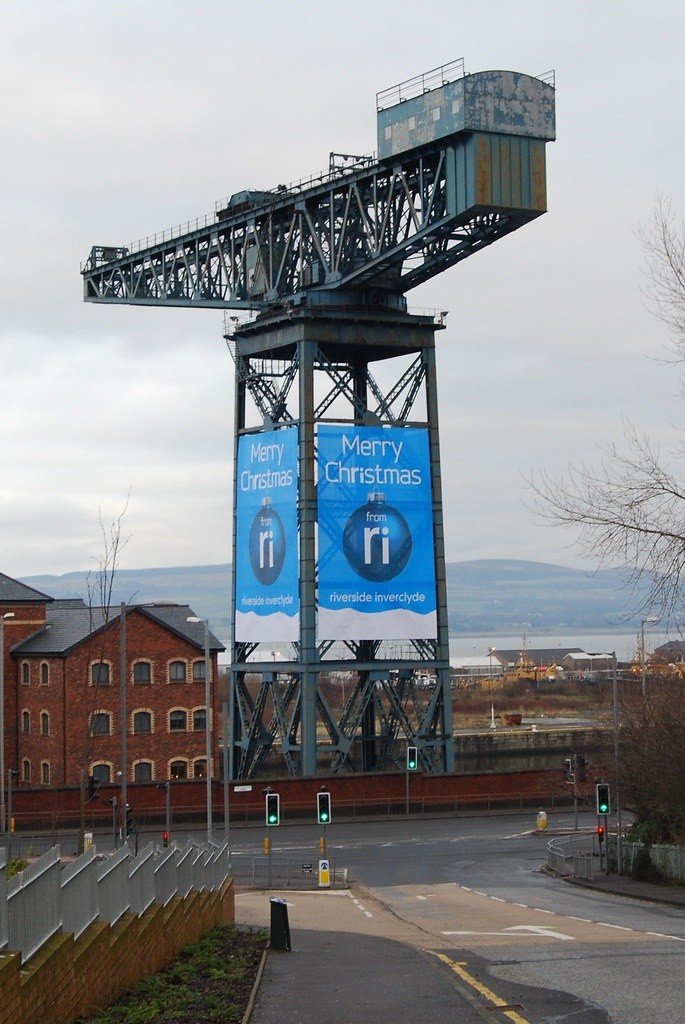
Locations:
[269,899,291,949]
[536,811,547,831]
[318,859,330,888]
[83,831,95,854]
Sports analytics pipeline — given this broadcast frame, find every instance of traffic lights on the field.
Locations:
[266,794,282,828]
[597,826,604,838]
[596,783,610,814]
[317,792,332,826]
[104,797,114,807]
[573,754,589,785]
[562,759,574,784]
[124,806,134,837]
[407,747,418,771]
[164,832,170,848]
[88,775,103,803]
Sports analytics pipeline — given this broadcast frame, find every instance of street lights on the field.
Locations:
[488,645,499,728]
[638,616,661,724]
[587,652,634,789]
